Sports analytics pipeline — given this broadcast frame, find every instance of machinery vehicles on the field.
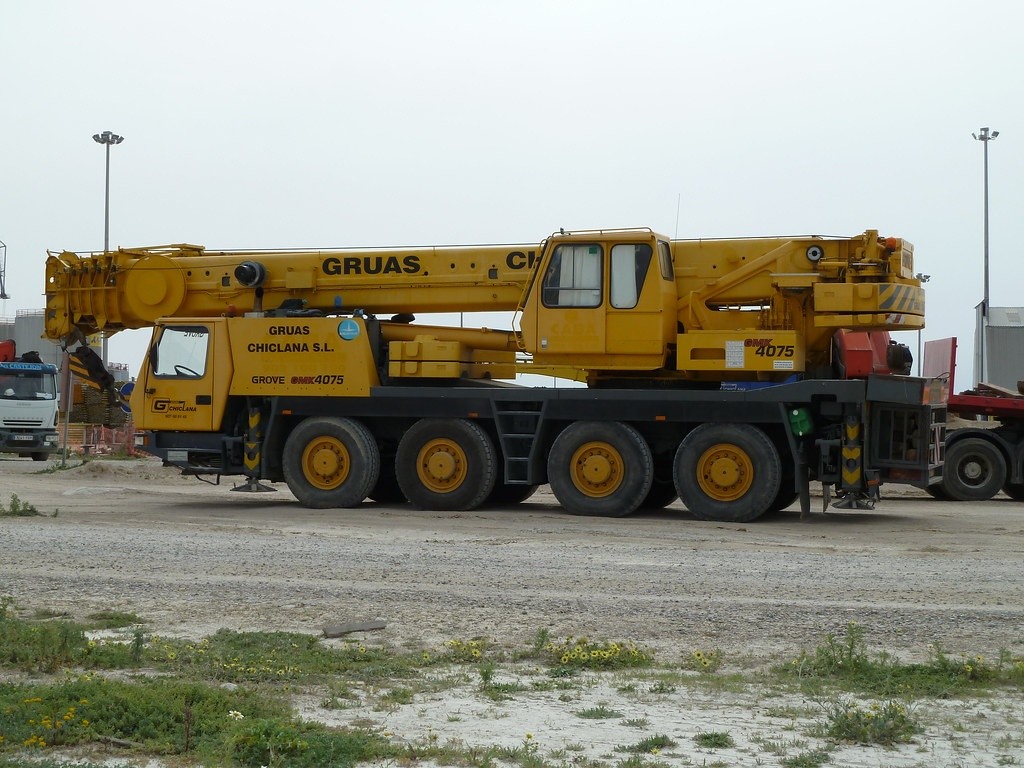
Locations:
[43,223,949,527]
[0,338,62,462]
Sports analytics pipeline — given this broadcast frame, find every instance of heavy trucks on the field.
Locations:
[838,327,1024,501]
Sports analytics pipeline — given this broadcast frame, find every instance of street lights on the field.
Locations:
[91,131,124,369]
[971,124,999,381]
